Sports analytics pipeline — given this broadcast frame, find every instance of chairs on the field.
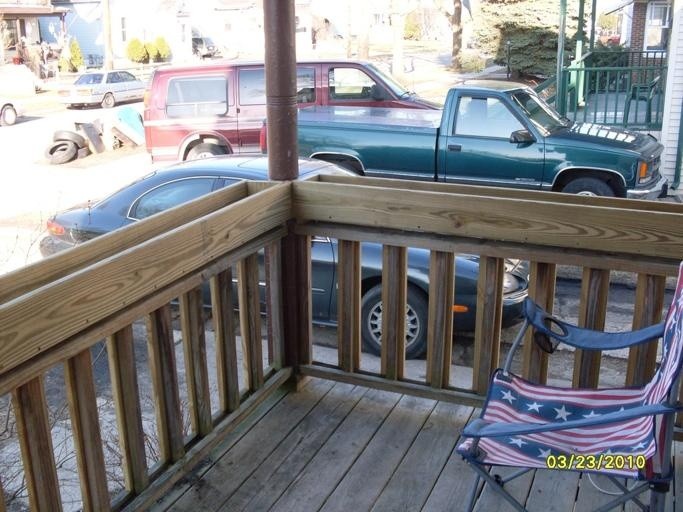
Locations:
[624,75,661,127]
[456,260,683,512]
[463,97,499,136]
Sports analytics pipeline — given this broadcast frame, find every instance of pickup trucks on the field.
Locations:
[296,79,669,199]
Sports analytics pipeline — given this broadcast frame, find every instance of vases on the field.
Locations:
[13,56,21,65]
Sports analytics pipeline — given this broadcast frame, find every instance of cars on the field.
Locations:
[0,64,36,125]
[58,69,147,109]
[39,152,530,361]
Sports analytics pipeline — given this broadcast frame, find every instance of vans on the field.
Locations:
[140,61,444,170]
